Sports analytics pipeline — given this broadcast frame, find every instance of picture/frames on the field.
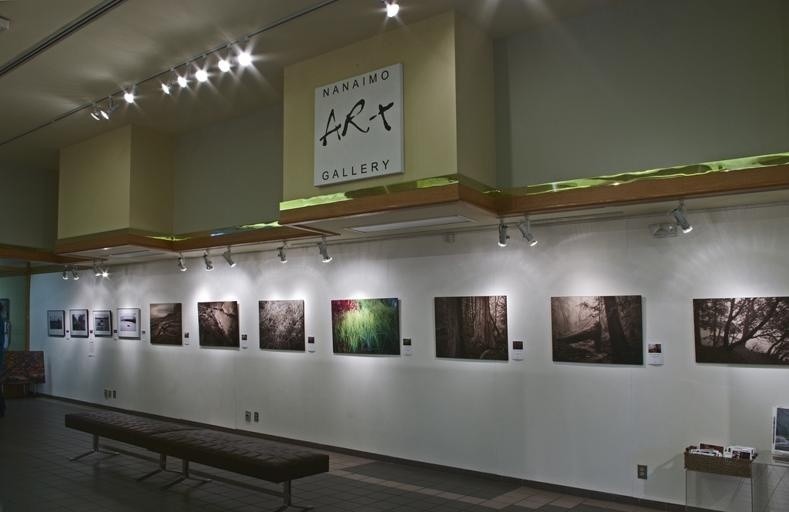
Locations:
[117,308,141,338]
[70,309,89,338]
[0,298,9,320]
[47,310,65,337]
[93,310,112,336]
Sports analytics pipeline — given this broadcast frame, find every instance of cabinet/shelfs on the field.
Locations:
[752,450,789,512]
[684,452,752,512]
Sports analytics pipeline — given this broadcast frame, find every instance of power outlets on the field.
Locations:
[638,465,647,479]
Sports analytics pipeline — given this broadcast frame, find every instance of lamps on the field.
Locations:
[518,214,539,246]
[62,198,333,281]
[384,0,401,18]
[498,218,510,247]
[91,1,254,121]
[669,199,694,234]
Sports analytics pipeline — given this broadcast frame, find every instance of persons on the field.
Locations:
[0,304,6,418]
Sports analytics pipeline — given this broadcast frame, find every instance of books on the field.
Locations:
[770,405,788,459]
[689,442,758,461]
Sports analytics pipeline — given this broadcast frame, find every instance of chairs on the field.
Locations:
[0,350,45,398]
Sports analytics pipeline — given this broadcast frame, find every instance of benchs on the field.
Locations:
[65,410,183,481]
[151,428,329,512]
